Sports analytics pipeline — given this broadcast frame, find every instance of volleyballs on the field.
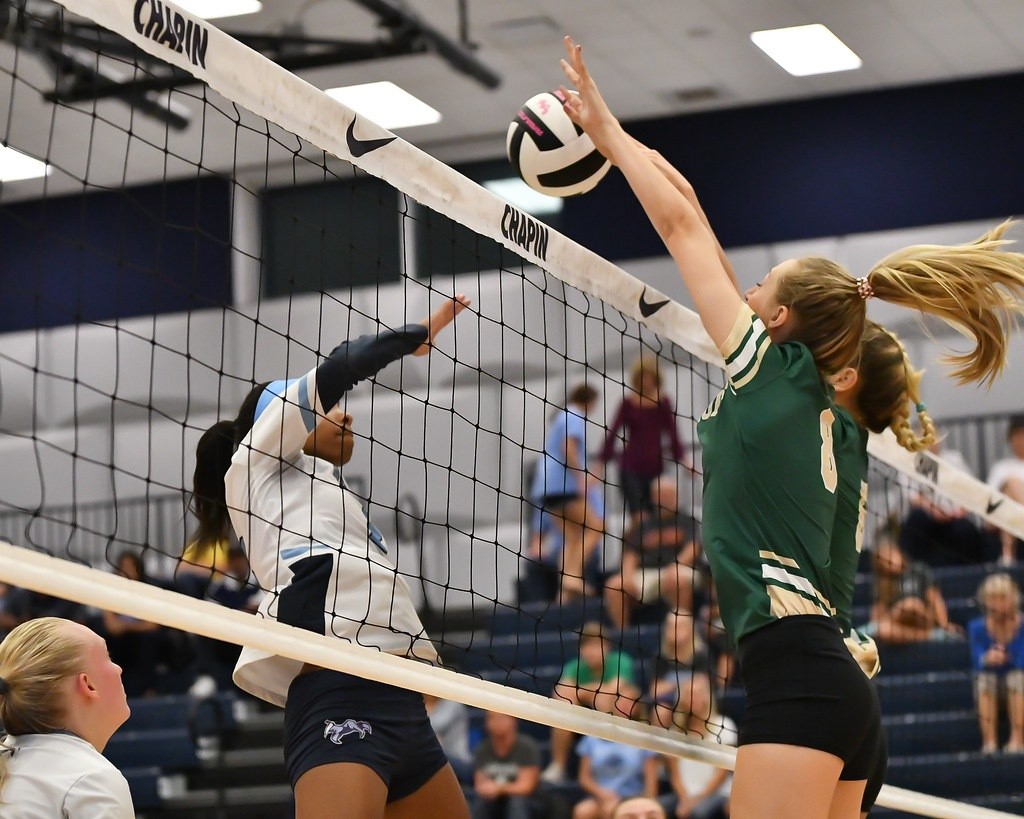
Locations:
[507,87,611,199]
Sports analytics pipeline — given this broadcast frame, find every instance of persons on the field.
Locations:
[1,36,1023,819]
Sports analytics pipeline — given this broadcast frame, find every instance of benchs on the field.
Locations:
[86,546,1024,819]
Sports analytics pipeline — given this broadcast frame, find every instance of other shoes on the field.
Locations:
[983,745,997,754]
[933,628,953,641]
[543,765,565,783]
[1004,744,1019,754]
